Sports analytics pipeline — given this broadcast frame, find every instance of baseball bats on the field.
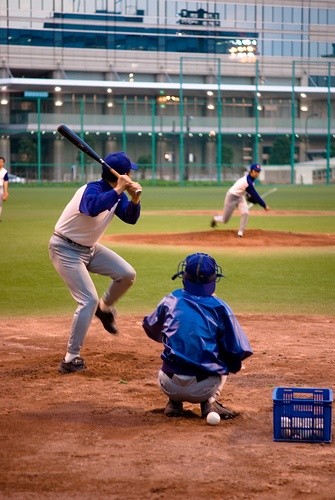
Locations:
[56,124,142,196]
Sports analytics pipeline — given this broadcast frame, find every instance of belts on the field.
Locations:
[54,231,91,249]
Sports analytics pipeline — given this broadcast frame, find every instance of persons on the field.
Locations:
[210,163,269,238]
[48,151,142,375]
[0,157,8,222]
[141,251,254,421]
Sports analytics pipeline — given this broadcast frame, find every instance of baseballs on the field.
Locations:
[205,411,221,426]
[280,415,323,439]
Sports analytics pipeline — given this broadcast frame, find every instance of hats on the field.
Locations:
[177,252,222,296]
[103,152,140,178]
[250,164,262,171]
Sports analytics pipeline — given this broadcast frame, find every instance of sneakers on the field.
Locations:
[238,230,244,236]
[58,358,87,376]
[162,401,184,417]
[211,220,216,227]
[94,304,119,335]
[202,400,238,420]
